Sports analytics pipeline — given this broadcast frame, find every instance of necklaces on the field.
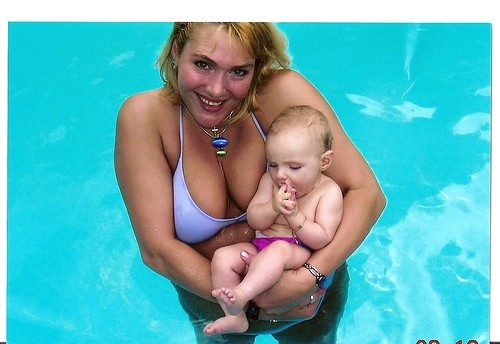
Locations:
[185,107,237,158]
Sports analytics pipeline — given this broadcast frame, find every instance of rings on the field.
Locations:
[310,296,315,305]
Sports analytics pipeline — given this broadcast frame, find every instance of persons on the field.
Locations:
[201,105,345,335]
[116,21,386,344]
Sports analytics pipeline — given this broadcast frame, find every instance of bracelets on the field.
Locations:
[303,263,327,288]
[293,216,307,236]
